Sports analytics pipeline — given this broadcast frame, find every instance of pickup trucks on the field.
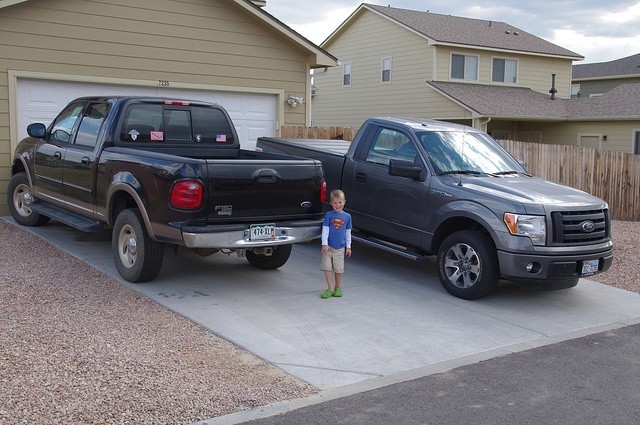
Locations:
[255,117,613,300]
[6,95,326,283]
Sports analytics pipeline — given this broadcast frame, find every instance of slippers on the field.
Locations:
[321,288,334,298]
[333,287,343,296]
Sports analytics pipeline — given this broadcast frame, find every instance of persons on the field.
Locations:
[320,190,353,299]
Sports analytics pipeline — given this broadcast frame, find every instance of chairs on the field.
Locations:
[128,124,154,139]
[163,125,190,139]
